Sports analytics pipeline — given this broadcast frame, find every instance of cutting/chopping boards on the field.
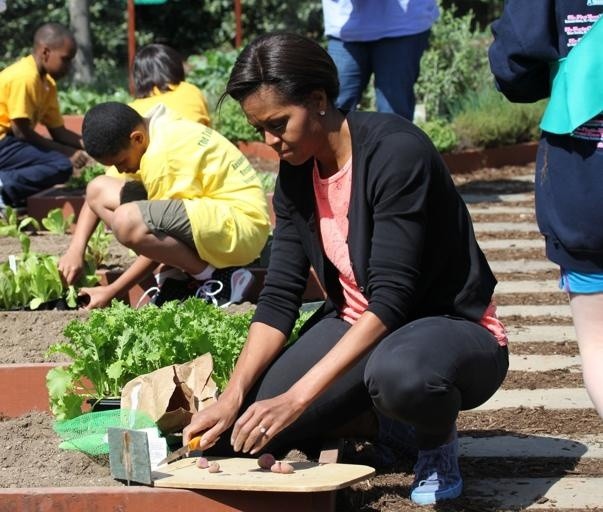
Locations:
[152,457,378,492]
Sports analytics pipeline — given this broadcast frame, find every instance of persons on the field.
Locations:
[54,100,271,313]
[106,41,211,182]
[0,21,90,209]
[486,0,602,421]
[320,1,442,124]
[180,30,510,507]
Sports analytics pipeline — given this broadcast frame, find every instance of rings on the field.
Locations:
[257,424,266,434]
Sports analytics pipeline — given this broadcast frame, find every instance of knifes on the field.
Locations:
[156,435,205,467]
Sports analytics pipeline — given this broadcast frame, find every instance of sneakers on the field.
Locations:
[135,270,201,307]
[196,265,257,310]
[0,178,29,214]
[359,405,412,466]
[409,422,463,506]
